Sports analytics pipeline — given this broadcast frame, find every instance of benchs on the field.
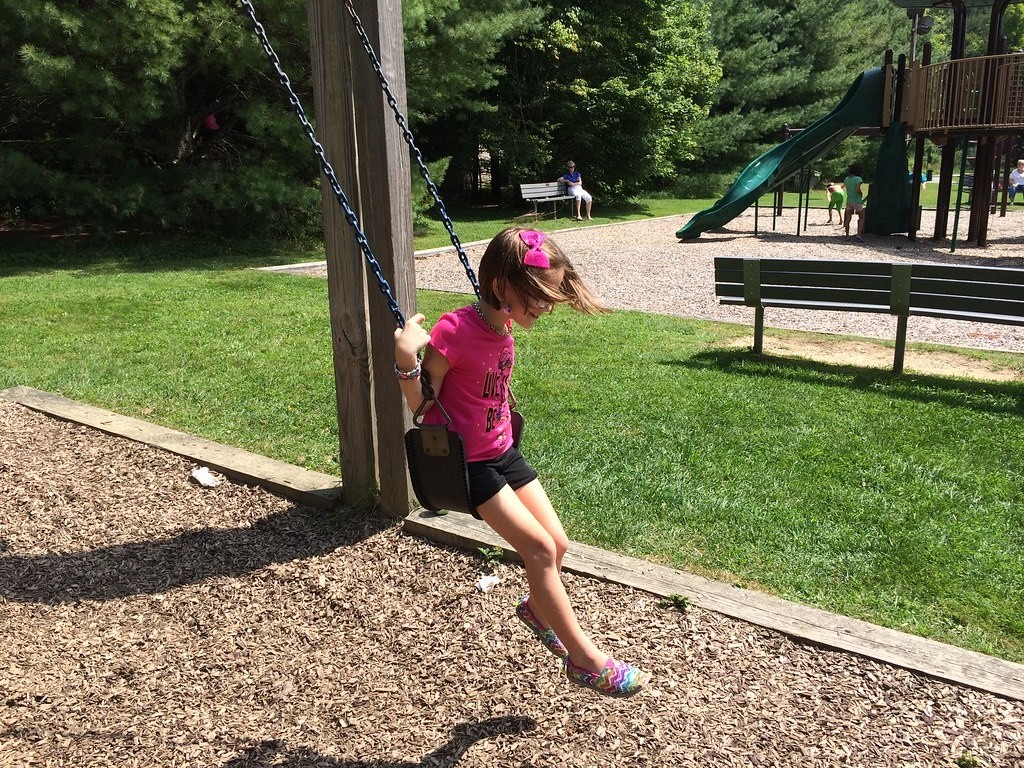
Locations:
[961,174,1023,204]
[714,256,1024,374]
[520,182,575,221]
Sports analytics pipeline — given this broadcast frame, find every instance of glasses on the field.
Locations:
[524,290,549,308]
[568,166,576,168]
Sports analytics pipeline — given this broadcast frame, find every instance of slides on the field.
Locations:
[674,64,885,240]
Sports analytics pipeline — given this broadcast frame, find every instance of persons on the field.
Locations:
[1009,159,1024,205]
[826,183,844,225]
[909,170,927,189]
[396,226,650,697]
[844,162,865,242]
[557,161,592,220]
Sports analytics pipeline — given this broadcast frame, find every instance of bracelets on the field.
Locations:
[394,359,422,381]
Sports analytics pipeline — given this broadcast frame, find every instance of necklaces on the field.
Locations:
[473,301,513,337]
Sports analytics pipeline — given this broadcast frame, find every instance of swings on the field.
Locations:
[238,0,528,522]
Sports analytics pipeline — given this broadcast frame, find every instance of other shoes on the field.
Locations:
[566,654,650,698]
[515,594,569,659]
